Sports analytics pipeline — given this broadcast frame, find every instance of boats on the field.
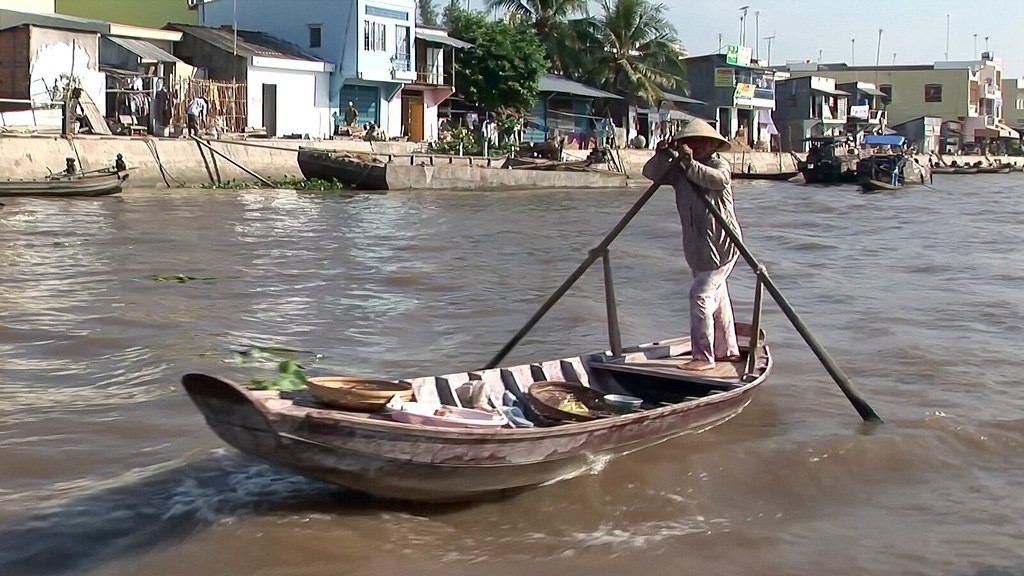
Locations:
[500,151,597,172]
[0,166,142,198]
[181,321,775,512]
[788,105,1024,197]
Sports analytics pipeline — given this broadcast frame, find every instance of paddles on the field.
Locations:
[881,165,938,192]
[656,142,885,425]
[482,146,691,370]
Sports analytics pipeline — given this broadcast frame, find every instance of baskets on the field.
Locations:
[306,376,414,414]
[527,380,620,425]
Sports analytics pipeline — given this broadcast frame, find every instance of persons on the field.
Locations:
[579,114,596,150]
[605,117,617,148]
[345,101,359,136]
[187,95,208,138]
[642,118,745,371]
[888,155,898,185]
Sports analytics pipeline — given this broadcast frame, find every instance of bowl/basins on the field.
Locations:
[384,400,509,430]
[603,393,643,408]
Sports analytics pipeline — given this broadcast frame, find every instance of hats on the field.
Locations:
[199,96,208,103]
[672,118,731,152]
